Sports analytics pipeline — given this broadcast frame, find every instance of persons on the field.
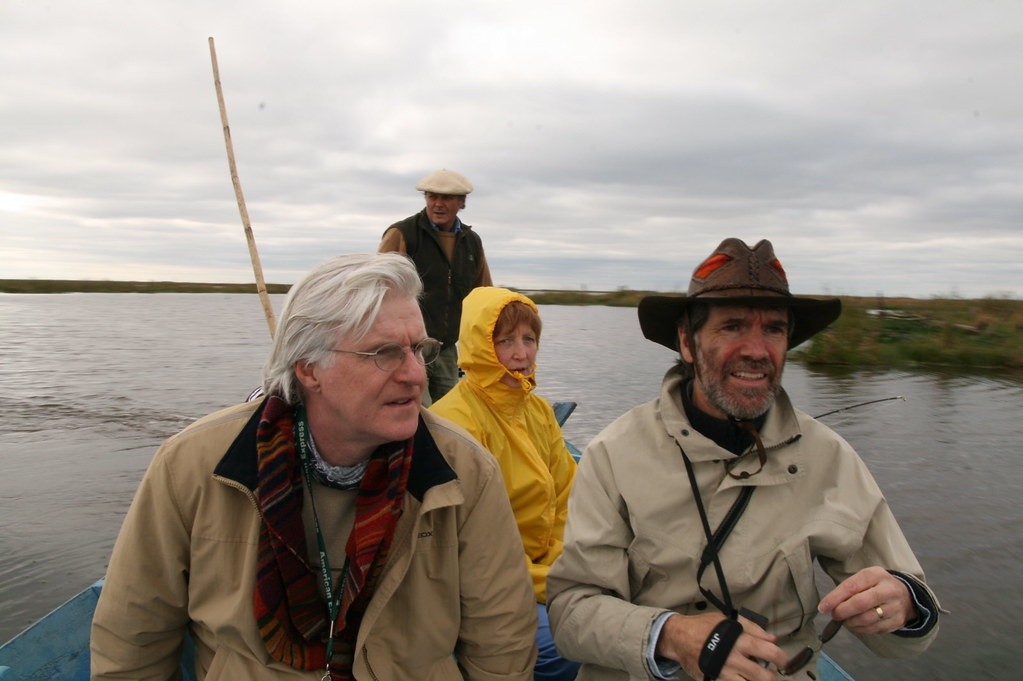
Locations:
[427,287,581,681]
[90,253,539,681]
[377,169,494,408]
[545,237,951,681]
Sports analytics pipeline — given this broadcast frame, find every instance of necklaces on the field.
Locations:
[306,429,365,489]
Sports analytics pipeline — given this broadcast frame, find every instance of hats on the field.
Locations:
[416,169,473,195]
[638,238,842,353]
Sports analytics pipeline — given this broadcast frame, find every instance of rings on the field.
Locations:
[875,606,884,619]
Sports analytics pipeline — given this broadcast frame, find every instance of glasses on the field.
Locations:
[328,339,444,372]
